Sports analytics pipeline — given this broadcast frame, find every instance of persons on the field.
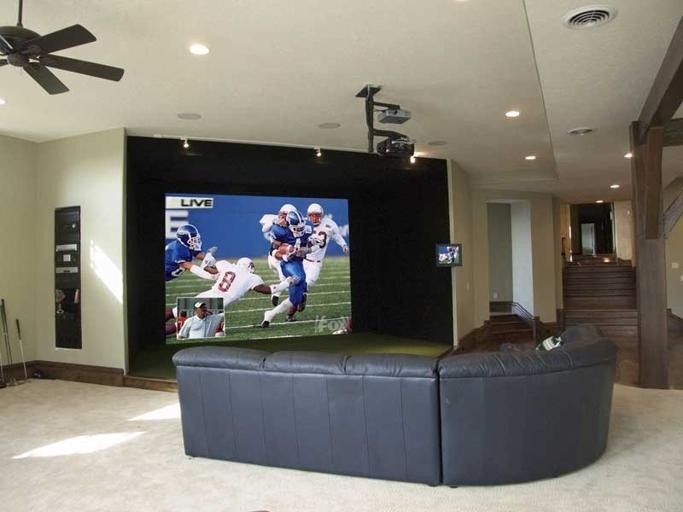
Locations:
[178,300,223,337]
[260,211,308,328]
[298,203,348,309]
[259,203,297,306]
[164,257,293,335]
[164,223,219,281]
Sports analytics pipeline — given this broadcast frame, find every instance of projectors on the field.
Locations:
[377,109,411,125]
[377,137,415,158]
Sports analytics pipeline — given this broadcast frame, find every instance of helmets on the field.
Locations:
[279,203,324,239]
[175,224,202,252]
[237,257,256,275]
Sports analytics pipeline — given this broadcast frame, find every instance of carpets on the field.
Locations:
[0,376,681,511]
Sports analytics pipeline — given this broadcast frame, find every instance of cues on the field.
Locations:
[0,298,28,389]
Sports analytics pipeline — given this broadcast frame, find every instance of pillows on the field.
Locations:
[537,334,562,351]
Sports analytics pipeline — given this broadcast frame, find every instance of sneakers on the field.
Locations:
[261,292,308,327]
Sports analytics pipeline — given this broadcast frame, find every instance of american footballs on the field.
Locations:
[277,246,298,254]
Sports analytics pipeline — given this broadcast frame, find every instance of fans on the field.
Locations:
[0,0,124,95]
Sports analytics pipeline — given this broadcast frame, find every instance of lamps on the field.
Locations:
[180,137,190,149]
[313,146,322,158]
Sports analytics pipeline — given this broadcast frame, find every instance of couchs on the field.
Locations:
[440,321,621,488]
[169,343,440,487]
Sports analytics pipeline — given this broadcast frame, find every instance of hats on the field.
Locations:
[194,301,206,308]
